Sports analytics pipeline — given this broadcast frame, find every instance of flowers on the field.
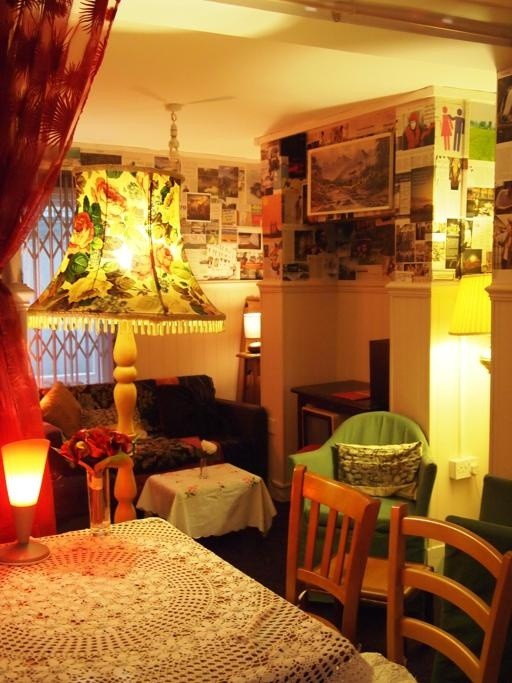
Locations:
[50,422,143,526]
[192,439,221,476]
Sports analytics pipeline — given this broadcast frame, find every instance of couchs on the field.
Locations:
[39,375,268,488]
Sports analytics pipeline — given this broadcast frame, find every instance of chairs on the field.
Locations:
[442,472,511,609]
[286,412,439,564]
[285,460,381,650]
[385,503,511,682]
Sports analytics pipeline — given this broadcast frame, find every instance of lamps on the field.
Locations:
[0,438,51,566]
[244,312,261,353]
[26,165,226,523]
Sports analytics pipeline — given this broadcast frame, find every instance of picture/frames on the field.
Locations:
[306,130,395,216]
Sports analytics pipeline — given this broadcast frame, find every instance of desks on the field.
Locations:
[306,547,434,647]
[136,462,277,539]
[0,517,375,683]
[237,352,260,405]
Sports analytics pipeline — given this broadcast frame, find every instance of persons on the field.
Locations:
[305,227,329,254]
[400,111,435,150]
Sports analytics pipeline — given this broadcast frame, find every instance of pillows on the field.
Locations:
[40,380,82,439]
[80,397,147,439]
[333,438,422,505]
[155,383,211,434]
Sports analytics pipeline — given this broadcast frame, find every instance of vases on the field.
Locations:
[200,459,207,477]
[86,469,111,537]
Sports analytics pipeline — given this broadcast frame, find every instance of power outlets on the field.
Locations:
[449,456,479,481]
